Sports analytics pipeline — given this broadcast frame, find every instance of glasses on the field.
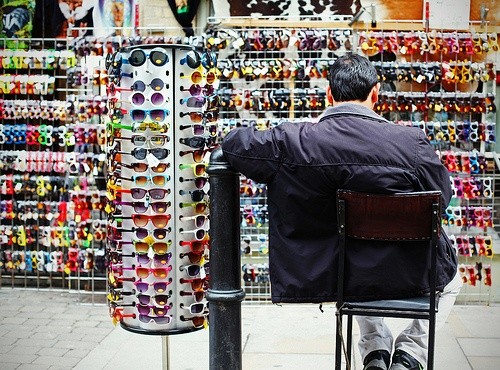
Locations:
[0,28,499,325]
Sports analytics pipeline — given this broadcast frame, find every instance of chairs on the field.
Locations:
[333,183,440,370]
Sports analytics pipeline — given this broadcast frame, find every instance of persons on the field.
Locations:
[221,50,464,370]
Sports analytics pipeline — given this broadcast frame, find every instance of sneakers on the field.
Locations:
[362,350,390,370]
[390,349,424,370]
